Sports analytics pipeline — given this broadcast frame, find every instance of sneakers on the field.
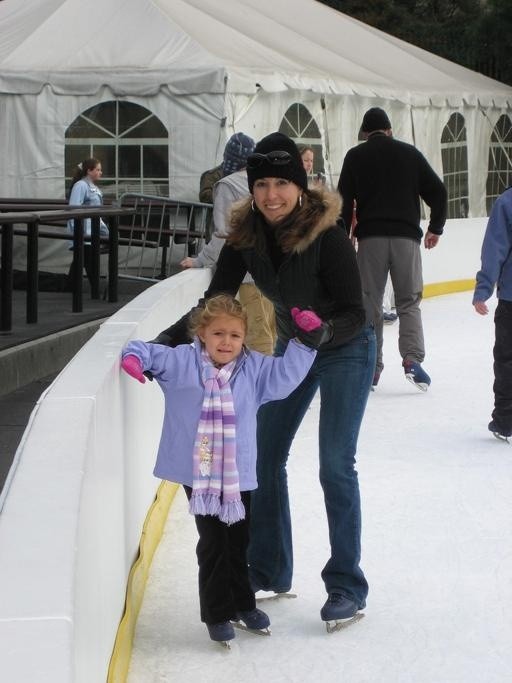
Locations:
[206,620,236,642]
[320,593,366,621]
[247,572,292,594]
[488,419,512,437]
[402,360,431,385]
[233,607,270,630]
[373,372,380,385]
[383,312,397,320]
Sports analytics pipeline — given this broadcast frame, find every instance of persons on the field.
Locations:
[120,291,323,643]
[63,157,111,291]
[299,144,314,173]
[471,183,511,439]
[336,107,449,388]
[347,197,398,321]
[198,149,228,244]
[179,130,278,356]
[140,132,380,624]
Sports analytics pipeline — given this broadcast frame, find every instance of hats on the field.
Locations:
[357,107,391,141]
[222,132,254,175]
[246,132,307,193]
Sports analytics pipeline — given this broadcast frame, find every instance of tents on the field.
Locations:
[0,1,510,293]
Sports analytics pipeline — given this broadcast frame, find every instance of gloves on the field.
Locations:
[295,306,334,348]
[122,354,146,384]
[291,307,323,332]
[143,332,175,381]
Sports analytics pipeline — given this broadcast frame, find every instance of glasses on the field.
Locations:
[245,151,294,171]
